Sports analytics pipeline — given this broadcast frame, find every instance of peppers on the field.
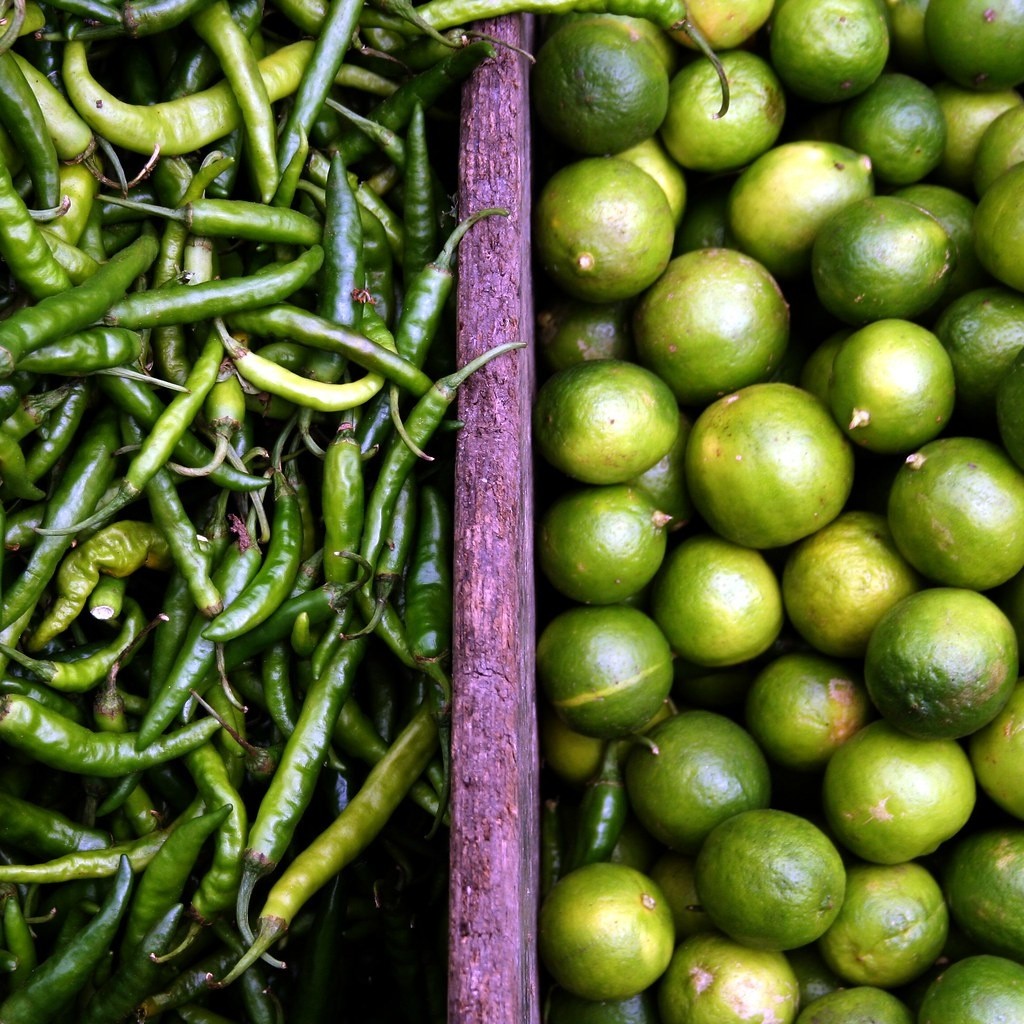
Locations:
[0,0,687,1024]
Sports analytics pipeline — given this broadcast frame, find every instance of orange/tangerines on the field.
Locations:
[535,0,1024,1024]
